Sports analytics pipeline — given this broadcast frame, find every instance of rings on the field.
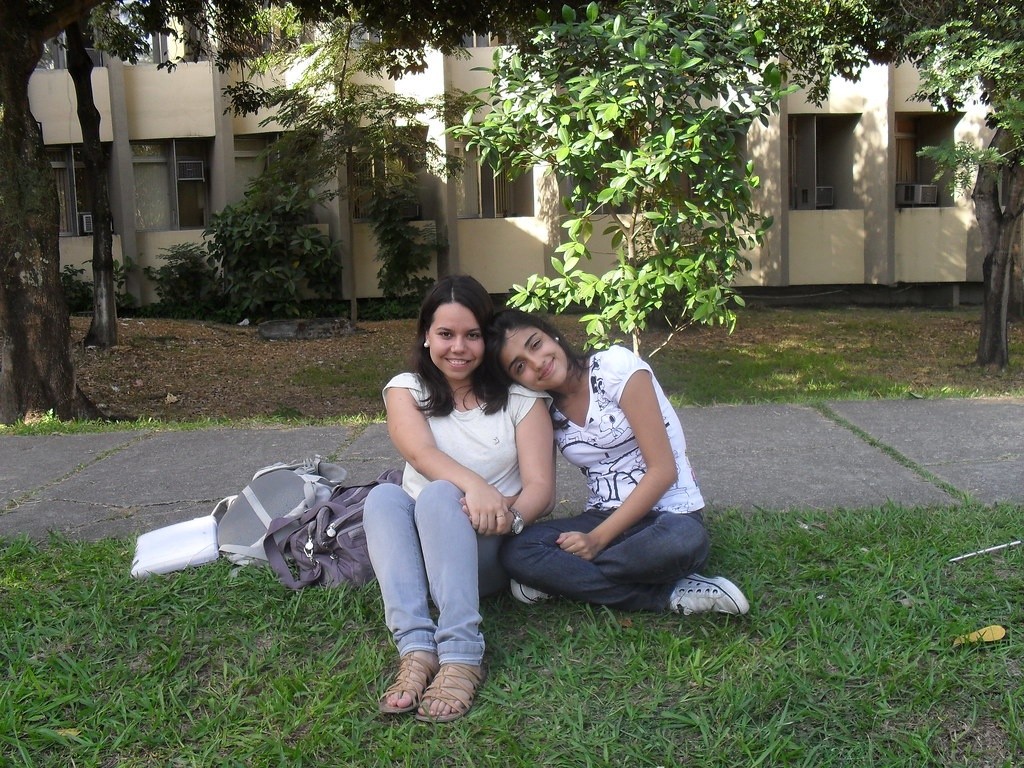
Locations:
[573,553,574,555]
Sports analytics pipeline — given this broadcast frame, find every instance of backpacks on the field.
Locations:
[212,458,346,567]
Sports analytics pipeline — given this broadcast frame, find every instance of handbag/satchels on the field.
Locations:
[263,468,404,587]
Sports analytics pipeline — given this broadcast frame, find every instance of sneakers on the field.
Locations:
[510,578,549,604]
[670,573,750,615]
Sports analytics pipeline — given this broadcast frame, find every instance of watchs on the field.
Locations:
[508,508,524,535]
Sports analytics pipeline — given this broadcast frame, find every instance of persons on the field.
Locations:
[493,311,749,615]
[362,275,553,722]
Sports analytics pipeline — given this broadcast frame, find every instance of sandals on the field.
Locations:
[415,663,486,722]
[378,651,438,714]
